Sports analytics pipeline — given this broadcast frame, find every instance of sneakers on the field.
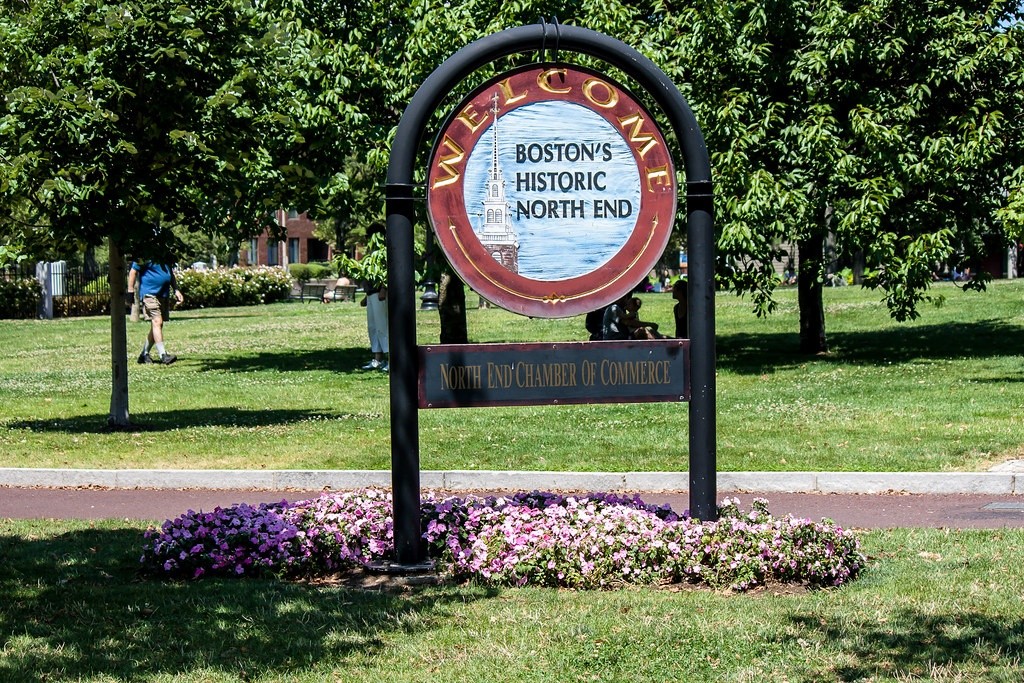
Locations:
[160,352,177,364]
[137,353,154,364]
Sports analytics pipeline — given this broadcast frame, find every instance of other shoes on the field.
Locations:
[362,359,381,369]
[380,363,389,371]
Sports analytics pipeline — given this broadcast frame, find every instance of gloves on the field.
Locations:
[126,292,135,304]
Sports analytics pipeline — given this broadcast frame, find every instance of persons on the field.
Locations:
[127,226,184,364]
[603,276,689,340]
[353,220,390,372]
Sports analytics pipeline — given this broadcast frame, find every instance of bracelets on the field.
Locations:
[380,287,387,290]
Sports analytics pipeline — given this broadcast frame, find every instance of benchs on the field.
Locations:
[325,285,357,304]
[287,283,328,304]
